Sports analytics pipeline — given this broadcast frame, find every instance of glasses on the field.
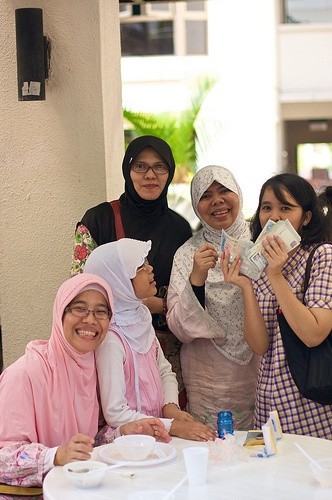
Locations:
[64,306,112,319]
[130,163,170,174]
[136,259,148,273]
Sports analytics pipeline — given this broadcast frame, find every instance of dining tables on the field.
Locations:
[43,430,332,500]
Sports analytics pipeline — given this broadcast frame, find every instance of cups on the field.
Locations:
[183,446,209,485]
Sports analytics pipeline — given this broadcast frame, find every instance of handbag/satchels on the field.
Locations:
[275,242,332,404]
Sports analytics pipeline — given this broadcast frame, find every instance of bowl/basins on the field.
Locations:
[114,434,155,460]
[63,461,108,488]
[310,458,332,485]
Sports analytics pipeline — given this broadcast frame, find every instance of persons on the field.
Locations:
[73,236,222,442]
[316,186,332,241]
[71,134,194,410]
[165,165,257,430]
[1,271,172,500]
[221,172,332,440]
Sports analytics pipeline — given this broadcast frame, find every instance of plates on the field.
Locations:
[100,442,176,467]
